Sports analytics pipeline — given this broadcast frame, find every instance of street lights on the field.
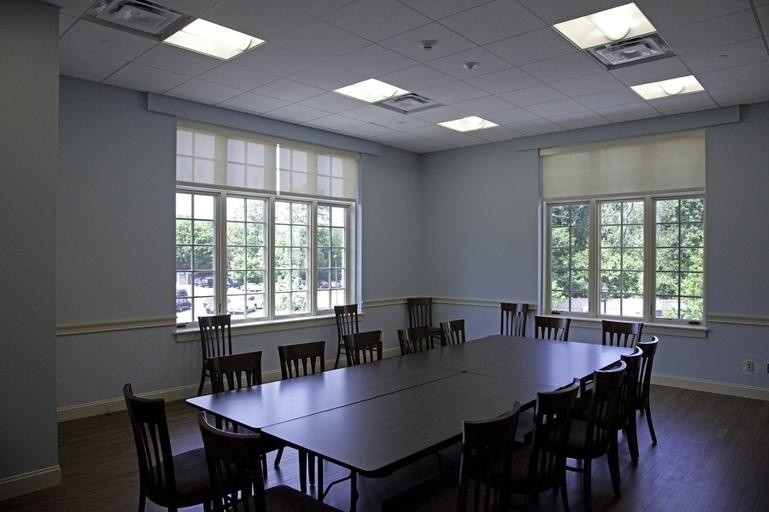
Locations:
[601,282,610,314]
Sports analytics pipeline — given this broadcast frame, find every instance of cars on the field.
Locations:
[177,271,342,314]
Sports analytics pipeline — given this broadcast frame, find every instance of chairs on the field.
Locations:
[198,296,658,512]
[125,382,207,512]
